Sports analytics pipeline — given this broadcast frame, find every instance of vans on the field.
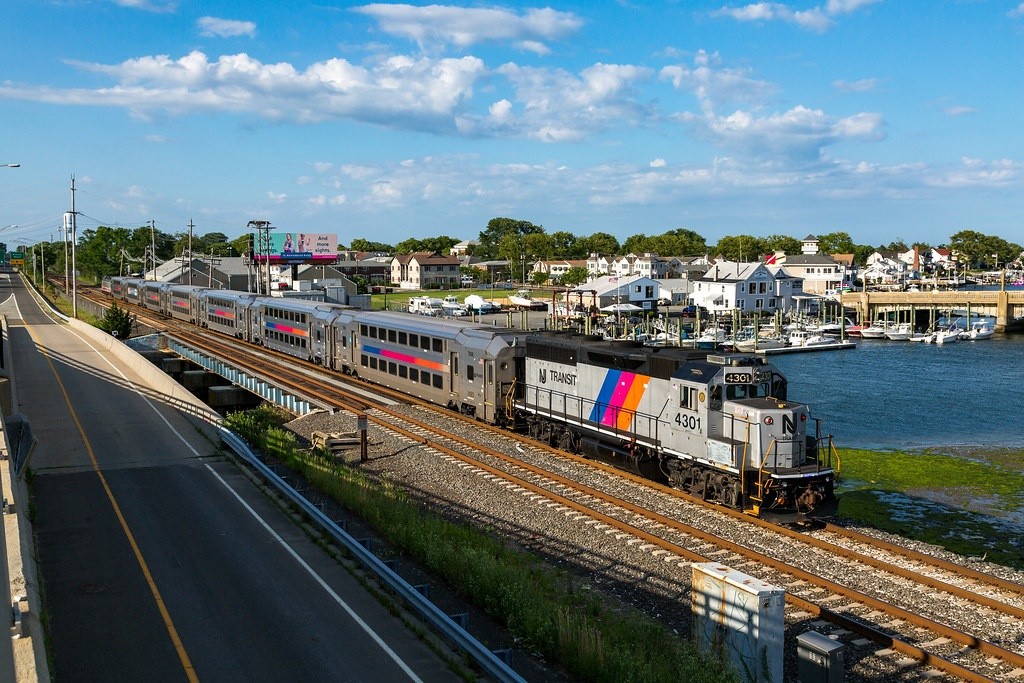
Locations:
[408,296,443,317]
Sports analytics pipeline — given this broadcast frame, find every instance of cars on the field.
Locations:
[480,304,502,313]
[444,304,467,317]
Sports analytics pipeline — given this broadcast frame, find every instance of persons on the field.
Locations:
[298,233,310,252]
[282,233,295,253]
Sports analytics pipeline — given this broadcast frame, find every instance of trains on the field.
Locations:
[101,274,842,524]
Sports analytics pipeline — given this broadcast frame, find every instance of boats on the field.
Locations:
[616,303,996,347]
